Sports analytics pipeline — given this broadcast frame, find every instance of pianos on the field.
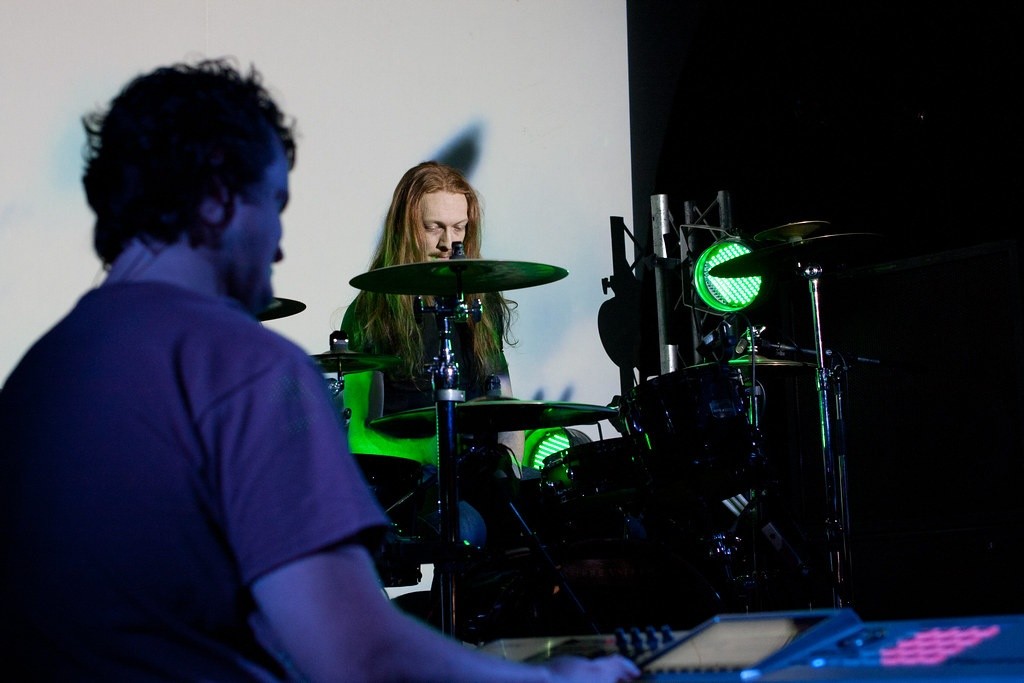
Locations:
[481,606,1024,680]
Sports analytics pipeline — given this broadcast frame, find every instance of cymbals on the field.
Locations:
[309,350,403,373]
[262,294,305,320]
[370,398,621,426]
[348,257,572,294]
[794,230,884,250]
[748,211,832,246]
[638,353,818,387]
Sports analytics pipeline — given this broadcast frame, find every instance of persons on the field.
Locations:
[0,61,640,683]
[341,161,524,579]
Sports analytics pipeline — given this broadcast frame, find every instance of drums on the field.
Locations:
[458,533,731,632]
[541,363,765,541]
